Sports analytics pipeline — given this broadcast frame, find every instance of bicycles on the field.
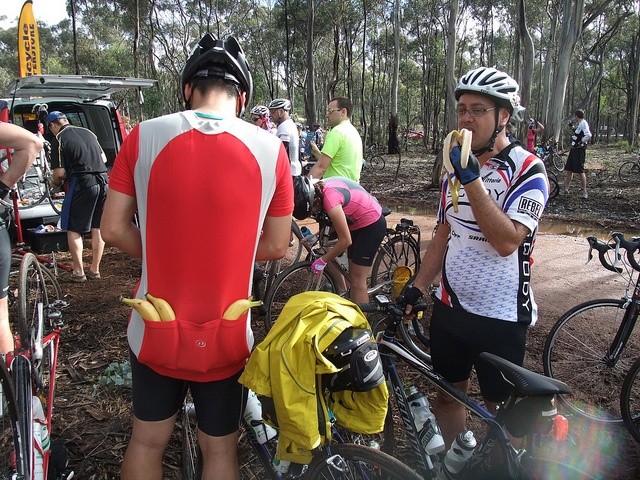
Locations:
[254,180,350,275]
[619,151,640,183]
[361,145,385,170]
[253,217,302,314]
[546,170,559,202]
[1,103,68,215]
[0,248,73,304]
[530,142,565,172]
[543,232,640,425]
[0,253,67,480]
[333,296,610,480]
[179,270,428,480]
[263,212,421,331]
[620,353,640,446]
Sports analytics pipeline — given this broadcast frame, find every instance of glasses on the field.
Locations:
[326,107,342,115]
[455,105,504,115]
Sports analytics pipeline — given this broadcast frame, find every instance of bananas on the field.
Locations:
[245,296,253,315]
[441,129,473,214]
[145,291,175,319]
[120,296,163,324]
[221,295,266,320]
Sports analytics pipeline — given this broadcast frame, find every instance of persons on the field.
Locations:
[249,105,277,139]
[398,65,550,480]
[558,109,593,199]
[267,96,304,179]
[292,173,387,319]
[0,120,43,415]
[312,124,324,151]
[303,125,314,155]
[44,110,111,283]
[294,122,310,176]
[527,117,544,156]
[98,31,294,480]
[305,96,363,185]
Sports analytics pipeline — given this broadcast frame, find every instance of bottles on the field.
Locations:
[22,397,49,454]
[242,387,276,444]
[271,447,289,478]
[407,385,445,455]
[301,226,313,241]
[14,417,43,480]
[302,128,312,154]
[445,432,478,473]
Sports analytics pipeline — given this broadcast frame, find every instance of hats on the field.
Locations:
[45,111,67,127]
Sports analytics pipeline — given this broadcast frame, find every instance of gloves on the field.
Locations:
[449,145,480,184]
[301,234,320,248]
[402,285,423,314]
[310,258,327,277]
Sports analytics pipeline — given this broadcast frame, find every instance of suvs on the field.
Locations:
[0,76,157,239]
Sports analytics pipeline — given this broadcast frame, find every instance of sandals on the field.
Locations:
[83,267,101,279]
[59,271,87,282]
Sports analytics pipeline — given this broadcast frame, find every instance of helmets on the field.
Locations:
[269,98,291,110]
[293,175,315,220]
[453,66,519,115]
[179,31,254,107]
[250,105,269,116]
